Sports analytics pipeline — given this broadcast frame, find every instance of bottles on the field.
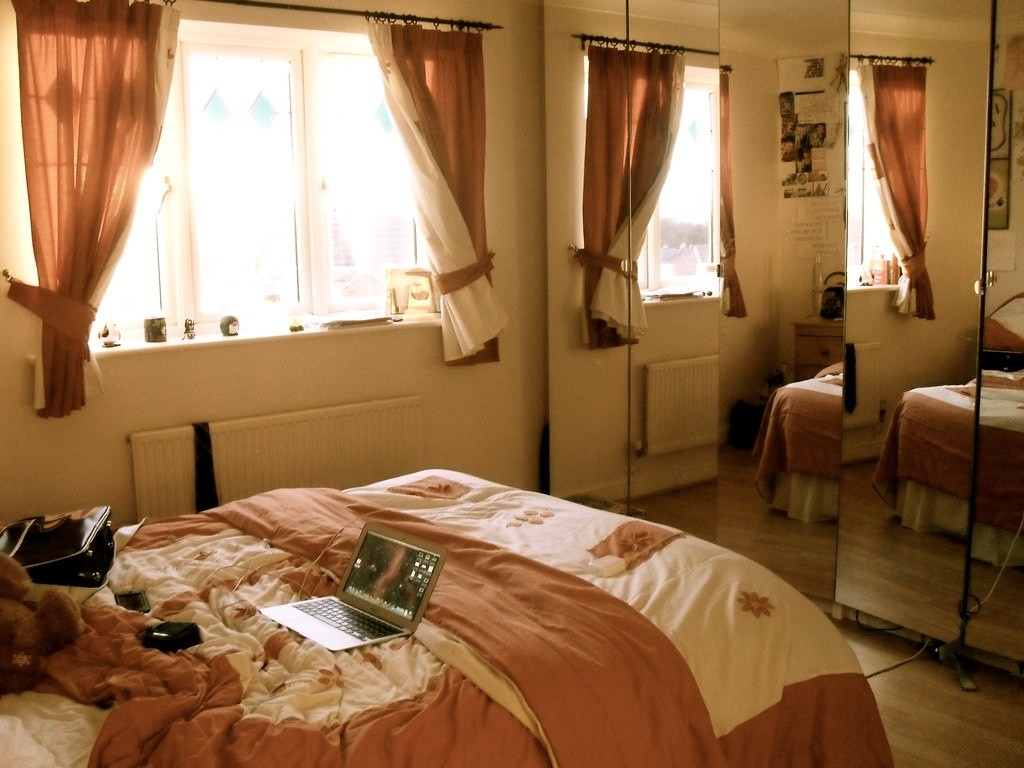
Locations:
[870,245,883,285]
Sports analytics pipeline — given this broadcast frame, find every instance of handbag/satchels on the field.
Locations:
[0,504,116,588]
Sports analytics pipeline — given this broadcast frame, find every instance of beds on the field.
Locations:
[0,468,892,767]
[748,362,844,523]
[871,378,977,539]
[970,369,1024,569]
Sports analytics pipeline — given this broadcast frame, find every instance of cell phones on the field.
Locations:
[115,590,150,613]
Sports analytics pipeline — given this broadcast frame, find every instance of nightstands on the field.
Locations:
[791,315,844,382]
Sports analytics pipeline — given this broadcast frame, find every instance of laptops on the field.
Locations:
[258,523,448,651]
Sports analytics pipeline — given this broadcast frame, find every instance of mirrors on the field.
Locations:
[544,0,1024,663]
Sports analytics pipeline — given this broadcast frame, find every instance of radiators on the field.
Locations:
[638,354,717,455]
[844,341,881,429]
[128,396,424,524]
